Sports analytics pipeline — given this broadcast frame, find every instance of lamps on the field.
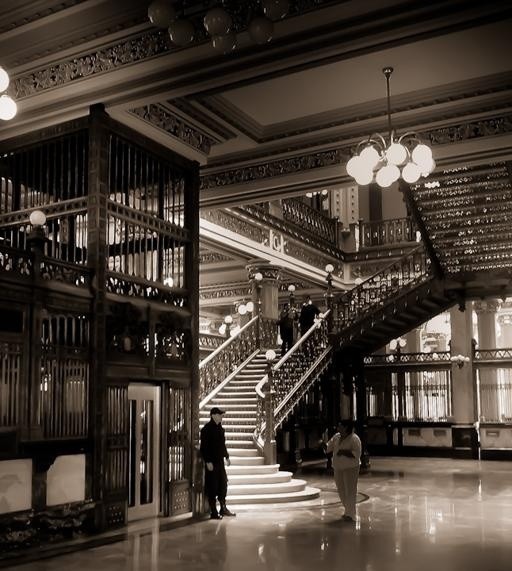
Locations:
[254,273,263,292]
[28,211,48,247]
[449,354,470,370]
[324,265,334,291]
[263,349,277,381]
[347,65,435,187]
[288,285,296,306]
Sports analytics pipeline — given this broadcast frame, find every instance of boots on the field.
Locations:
[208,500,222,519]
[220,500,236,516]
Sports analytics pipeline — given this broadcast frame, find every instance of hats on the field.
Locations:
[210,408,225,416]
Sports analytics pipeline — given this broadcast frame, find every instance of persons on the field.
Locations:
[297,294,321,358]
[199,407,236,519]
[279,301,298,357]
[319,417,362,522]
